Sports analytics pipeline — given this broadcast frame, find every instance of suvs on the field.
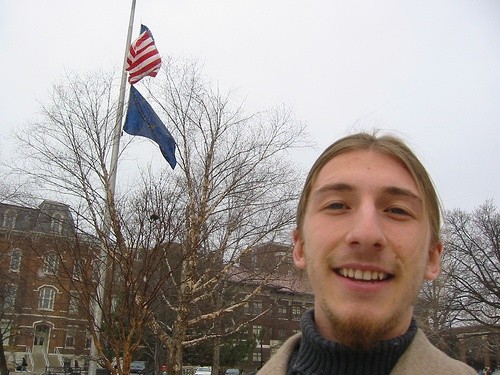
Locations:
[194,364,212,375]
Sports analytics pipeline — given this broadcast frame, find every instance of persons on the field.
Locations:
[21,355,28,373]
[254,132,480,375]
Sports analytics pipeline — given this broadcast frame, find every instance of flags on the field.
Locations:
[123,83,177,170]
[124,23,162,85]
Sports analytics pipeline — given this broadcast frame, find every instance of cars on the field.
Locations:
[224,367,243,375]
[129,360,147,374]
